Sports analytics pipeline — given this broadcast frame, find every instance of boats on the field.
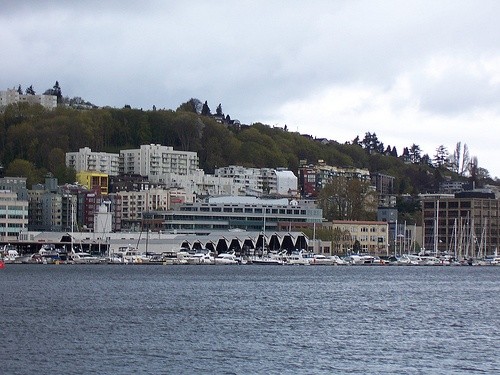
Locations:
[2,245,500,267]
